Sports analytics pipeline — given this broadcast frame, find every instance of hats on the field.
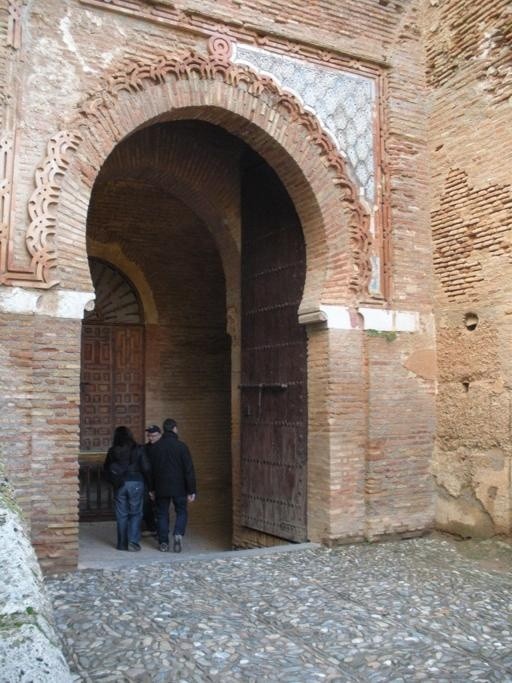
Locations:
[145,425,162,434]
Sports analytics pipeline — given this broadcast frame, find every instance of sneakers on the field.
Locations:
[159,535,183,553]
[117,541,142,552]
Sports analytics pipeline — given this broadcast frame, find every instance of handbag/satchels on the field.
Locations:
[104,459,127,486]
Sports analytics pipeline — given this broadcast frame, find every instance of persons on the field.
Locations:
[147,417,198,552]
[103,425,158,553]
[138,424,162,536]
